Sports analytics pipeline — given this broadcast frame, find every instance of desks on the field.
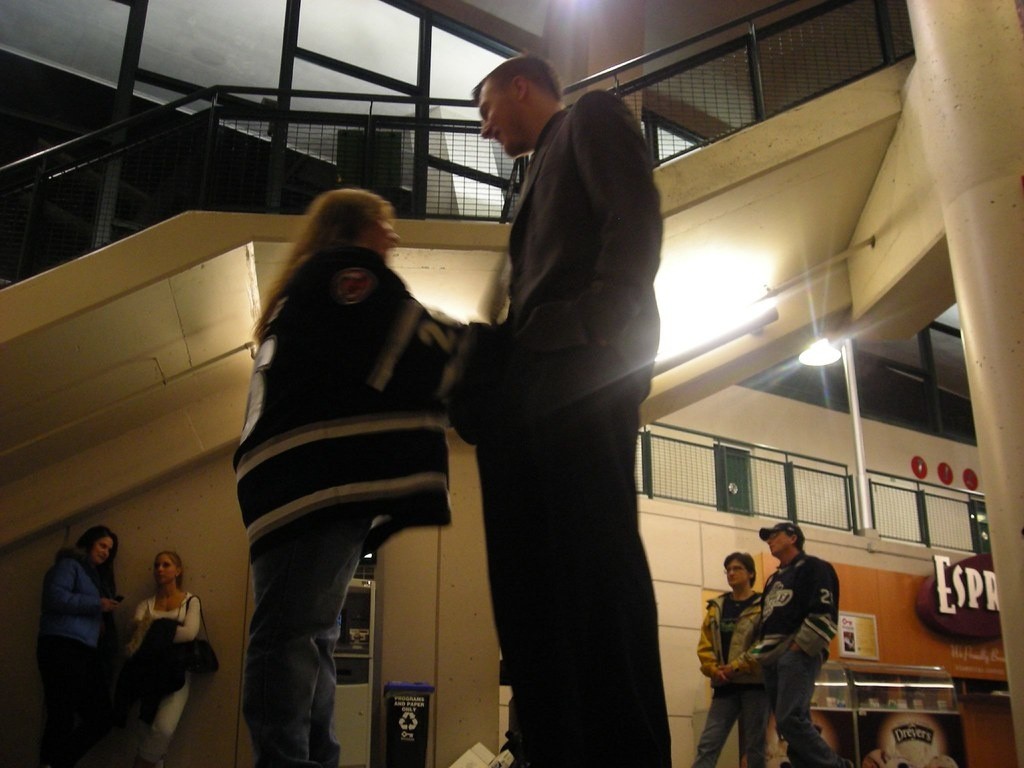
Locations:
[820,664,1017,768]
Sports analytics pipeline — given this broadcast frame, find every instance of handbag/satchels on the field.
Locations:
[182,595,218,674]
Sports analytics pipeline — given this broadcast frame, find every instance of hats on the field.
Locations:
[759,522,805,548]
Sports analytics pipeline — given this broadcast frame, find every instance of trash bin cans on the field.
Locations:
[383,681,435,768]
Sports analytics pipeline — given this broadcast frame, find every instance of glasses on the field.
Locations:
[769,530,781,539]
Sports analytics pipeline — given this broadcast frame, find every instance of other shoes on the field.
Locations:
[844,759,853,768]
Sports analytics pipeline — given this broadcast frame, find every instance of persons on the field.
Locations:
[691,523,856,768]
[130,551,201,768]
[474,54,672,768]
[232,188,472,768]
[36,526,119,768]
